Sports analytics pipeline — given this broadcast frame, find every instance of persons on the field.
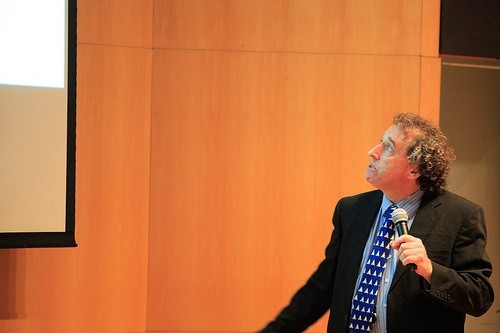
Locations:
[257,112,495,333]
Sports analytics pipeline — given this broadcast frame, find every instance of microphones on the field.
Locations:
[392,208,417,272]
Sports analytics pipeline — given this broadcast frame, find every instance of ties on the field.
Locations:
[347,203,399,332]
